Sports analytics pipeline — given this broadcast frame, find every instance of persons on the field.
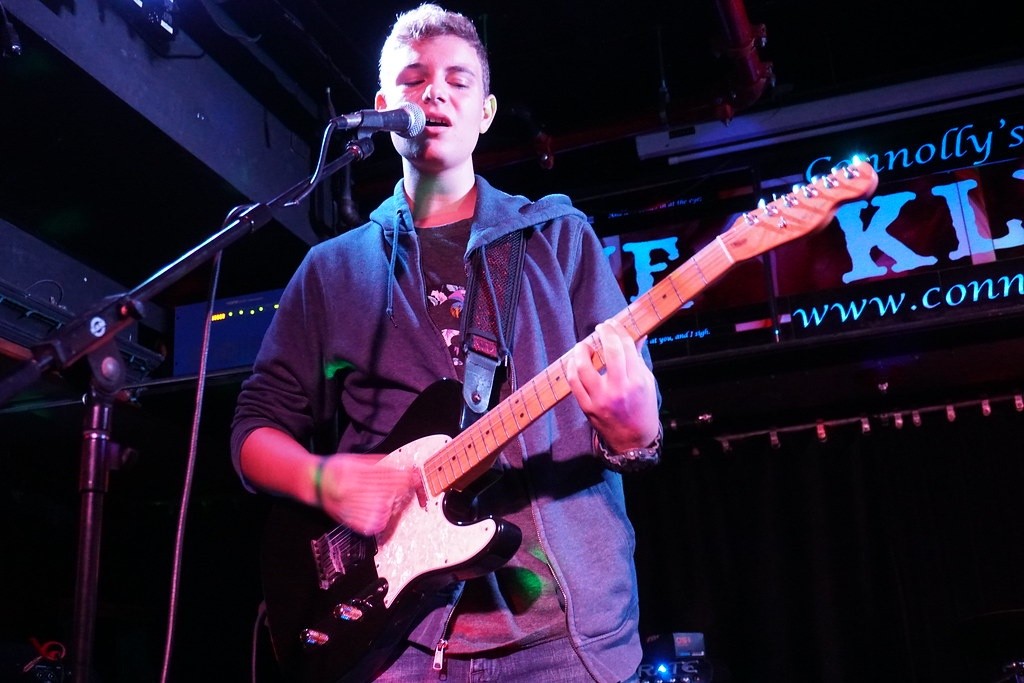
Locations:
[230,4,664,683]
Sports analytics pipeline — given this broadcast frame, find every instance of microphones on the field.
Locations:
[328,102,426,139]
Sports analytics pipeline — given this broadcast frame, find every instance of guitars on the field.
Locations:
[255,152,889,683]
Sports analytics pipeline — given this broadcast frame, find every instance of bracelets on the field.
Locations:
[316,454,328,511]
